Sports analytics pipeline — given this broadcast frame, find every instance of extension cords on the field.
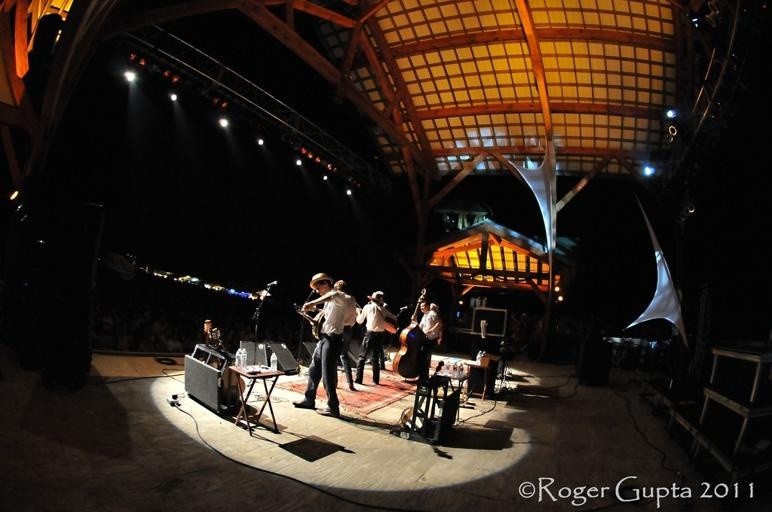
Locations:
[167,396,180,405]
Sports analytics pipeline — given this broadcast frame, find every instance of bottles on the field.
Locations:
[235,347,248,370]
[475,350,486,366]
[269,352,278,372]
[475,295,488,308]
[444,360,464,378]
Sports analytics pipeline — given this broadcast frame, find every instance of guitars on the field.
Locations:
[211,328,246,399]
[357,305,400,334]
[292,303,324,340]
[399,361,444,427]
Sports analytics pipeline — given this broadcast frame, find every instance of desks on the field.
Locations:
[228,365,286,437]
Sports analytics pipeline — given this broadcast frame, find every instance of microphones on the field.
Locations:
[267,280,280,286]
[399,306,410,310]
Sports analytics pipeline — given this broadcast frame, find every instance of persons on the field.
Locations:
[0,261,649,385]
[291,271,358,421]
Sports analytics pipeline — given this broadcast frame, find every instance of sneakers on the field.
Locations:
[355,379,362,384]
[317,408,339,417]
[350,384,356,391]
[293,400,315,410]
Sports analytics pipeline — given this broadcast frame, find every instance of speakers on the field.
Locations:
[297,341,317,367]
[266,342,299,371]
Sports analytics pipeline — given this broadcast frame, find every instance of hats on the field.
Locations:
[367,291,384,304]
[310,273,333,290]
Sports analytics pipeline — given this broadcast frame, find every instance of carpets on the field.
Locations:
[272,367,417,415]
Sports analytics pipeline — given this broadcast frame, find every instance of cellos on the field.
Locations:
[393,289,426,378]
